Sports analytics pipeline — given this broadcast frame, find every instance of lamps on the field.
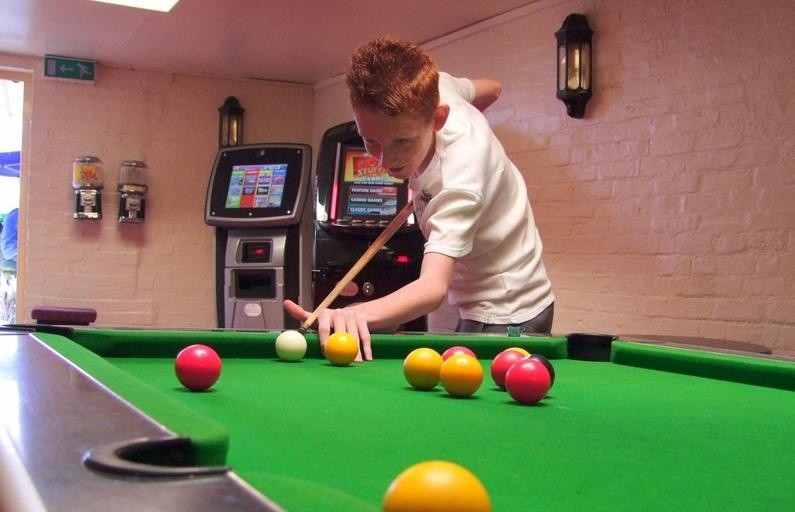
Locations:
[553,11,594,118]
[217,93,245,150]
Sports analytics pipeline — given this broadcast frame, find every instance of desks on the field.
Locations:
[0,327,794,511]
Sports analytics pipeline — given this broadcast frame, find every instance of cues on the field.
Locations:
[299,200,415,334]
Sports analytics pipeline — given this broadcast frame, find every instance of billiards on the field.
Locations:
[383,461,492,512]
[275,330,307,361]
[492,348,554,403]
[440,354,483,395]
[326,331,358,365]
[403,348,443,389]
[176,344,221,390]
[439,345,477,362]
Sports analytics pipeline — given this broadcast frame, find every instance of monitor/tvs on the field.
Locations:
[337,147,409,222]
[224,162,290,210]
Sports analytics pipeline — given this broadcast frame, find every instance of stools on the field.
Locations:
[30,304,99,327]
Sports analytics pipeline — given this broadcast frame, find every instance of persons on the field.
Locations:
[282,33,556,363]
